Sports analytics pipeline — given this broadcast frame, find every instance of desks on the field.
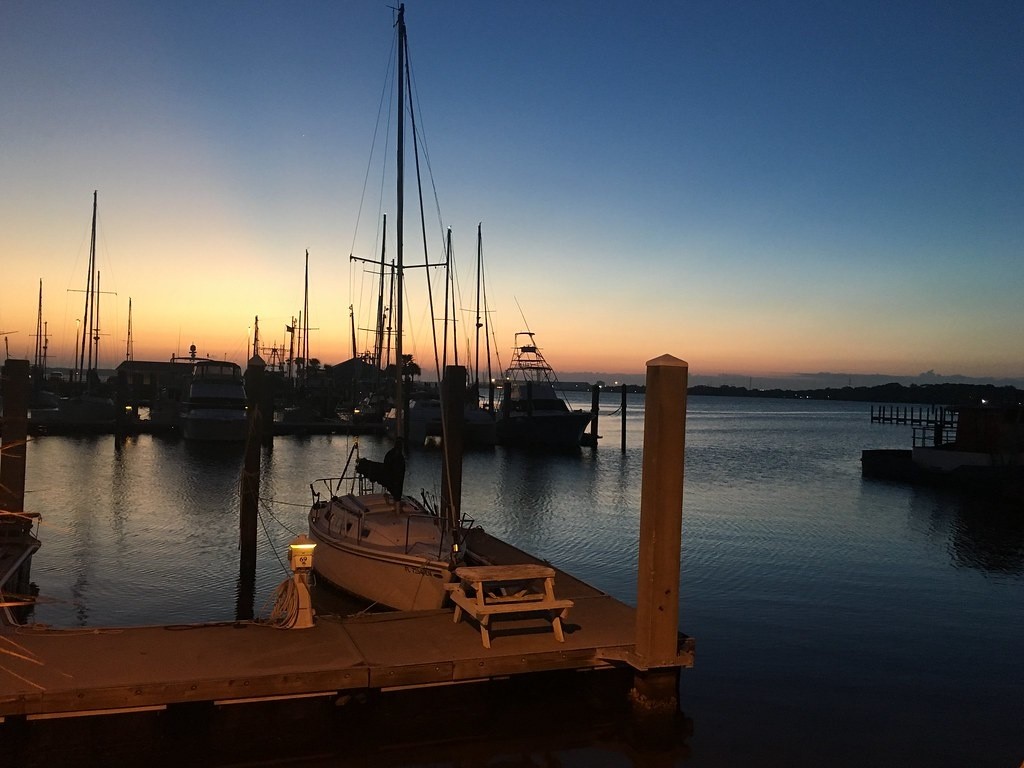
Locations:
[450,563,565,648]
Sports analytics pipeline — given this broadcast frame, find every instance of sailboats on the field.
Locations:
[257,0,511,613]
[0,1,599,449]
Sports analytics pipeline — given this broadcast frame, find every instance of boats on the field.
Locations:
[860,405,1024,490]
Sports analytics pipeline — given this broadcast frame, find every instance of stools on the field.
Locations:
[445,581,574,623]
